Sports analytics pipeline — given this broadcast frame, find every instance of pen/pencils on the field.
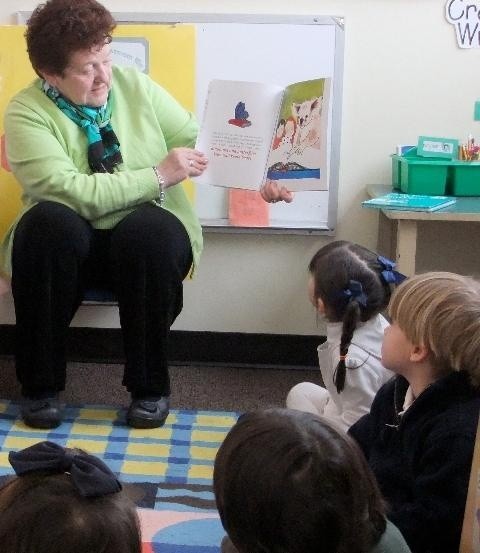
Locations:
[458,132,479,161]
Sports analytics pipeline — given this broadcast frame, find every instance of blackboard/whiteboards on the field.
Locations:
[12,11,346,236]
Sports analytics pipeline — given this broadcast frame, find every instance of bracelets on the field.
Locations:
[154,165,164,206]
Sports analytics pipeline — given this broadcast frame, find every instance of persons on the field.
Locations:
[0,440,143,551]
[283,242,407,435]
[212,404,413,553]
[2,0,296,426]
[342,269,479,552]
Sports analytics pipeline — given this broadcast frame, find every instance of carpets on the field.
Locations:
[0,396,247,552]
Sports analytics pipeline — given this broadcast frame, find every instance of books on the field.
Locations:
[360,191,457,213]
[188,77,329,192]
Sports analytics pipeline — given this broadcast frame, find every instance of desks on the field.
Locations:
[365,182,479,278]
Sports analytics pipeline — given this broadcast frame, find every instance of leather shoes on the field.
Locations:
[126,395,170,428]
[21,393,63,428]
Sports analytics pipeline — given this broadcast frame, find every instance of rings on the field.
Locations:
[272,199,277,203]
[189,158,194,166]
